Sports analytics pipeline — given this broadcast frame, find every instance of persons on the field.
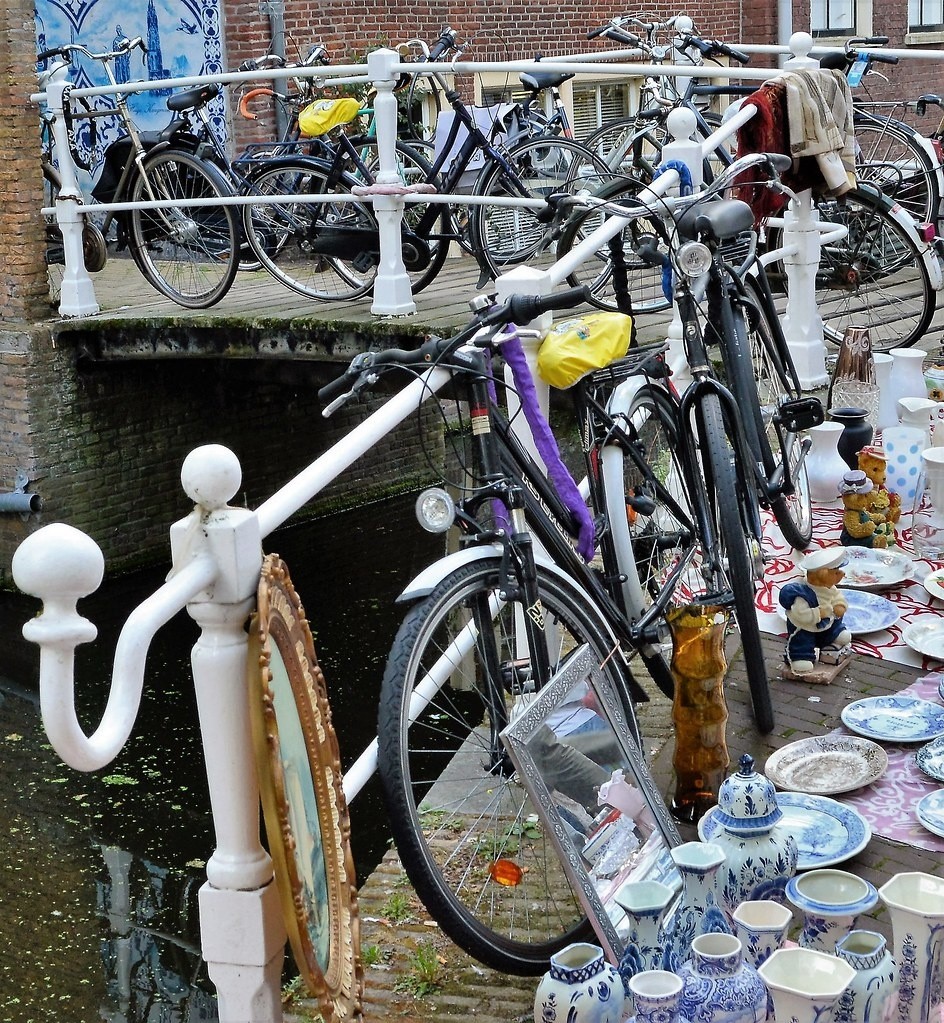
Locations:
[525,681,639,841]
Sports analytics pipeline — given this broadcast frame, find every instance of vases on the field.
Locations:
[872,353,898,434]
[827,408,874,471]
[533,841,944,1023]
[889,348,928,418]
[807,421,853,503]
[920,445,944,530]
[900,397,936,490]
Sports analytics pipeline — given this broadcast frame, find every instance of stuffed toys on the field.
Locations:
[837,446,901,548]
[779,546,854,674]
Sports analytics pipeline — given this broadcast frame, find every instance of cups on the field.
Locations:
[881,426,926,513]
[914,470,944,559]
[832,381,880,443]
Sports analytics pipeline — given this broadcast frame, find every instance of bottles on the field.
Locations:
[872,352,900,433]
[826,408,873,471]
[828,324,877,410]
[534,841,944,1023]
[807,422,852,503]
[921,446,944,530]
[889,348,927,418]
[896,396,936,448]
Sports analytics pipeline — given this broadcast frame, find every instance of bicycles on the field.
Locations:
[38,38,241,308]
[318,286,737,976]
[167,12,943,355]
[538,151,825,734]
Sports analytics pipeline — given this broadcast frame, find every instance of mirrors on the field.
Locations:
[501,642,684,970]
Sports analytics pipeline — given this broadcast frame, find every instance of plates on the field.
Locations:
[838,589,901,635]
[913,734,944,782]
[902,618,944,663]
[916,786,944,840]
[924,568,944,601]
[697,792,872,870]
[764,734,889,795]
[799,546,917,592]
[840,695,944,743]
